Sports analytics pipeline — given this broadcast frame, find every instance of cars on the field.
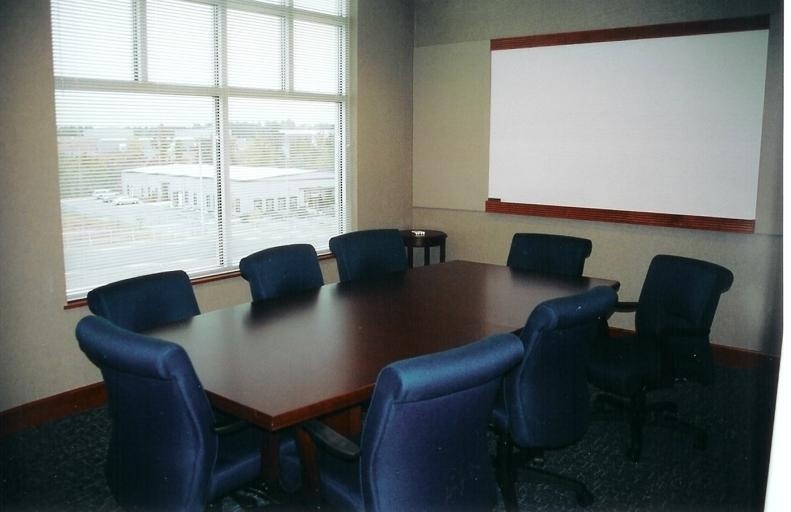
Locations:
[92,188,139,205]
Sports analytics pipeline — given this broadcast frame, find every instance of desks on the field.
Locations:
[127,260,621,512]
[399,229,448,269]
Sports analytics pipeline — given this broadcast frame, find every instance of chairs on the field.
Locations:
[238,244,324,304]
[507,233,592,278]
[75,316,263,512]
[87,269,200,330]
[484,284,620,512]
[329,229,410,282]
[607,255,735,462]
[302,331,529,512]
